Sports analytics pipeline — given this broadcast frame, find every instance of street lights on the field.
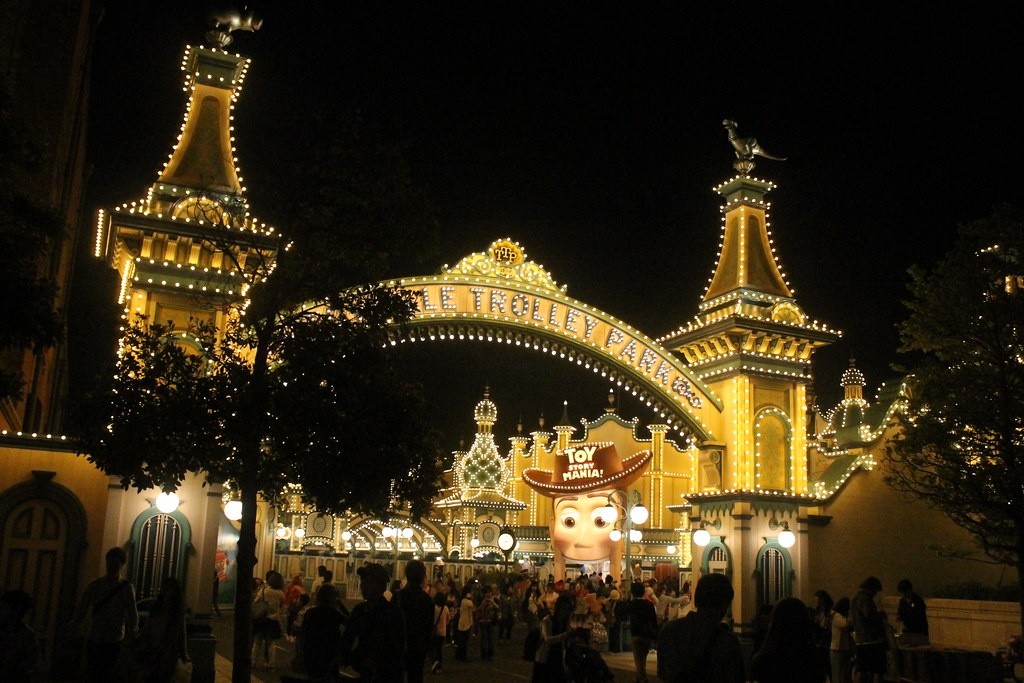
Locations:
[381,524,414,580]
[599,488,649,602]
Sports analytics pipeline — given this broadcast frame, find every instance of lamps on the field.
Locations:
[769,517,795,548]
[667,528,679,555]
[693,517,722,546]
[340,518,351,541]
[294,515,305,537]
[223,486,243,520]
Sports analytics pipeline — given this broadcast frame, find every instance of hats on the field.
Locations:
[356,562,390,588]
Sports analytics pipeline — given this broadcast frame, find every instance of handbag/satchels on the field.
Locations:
[431,626,437,636]
[291,655,305,674]
[523,629,541,661]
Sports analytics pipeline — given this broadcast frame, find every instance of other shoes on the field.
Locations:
[264,665,274,672]
[454,644,458,647]
[497,636,503,640]
[472,634,477,640]
[506,635,511,639]
[480,654,487,662]
[487,653,497,665]
[431,661,442,674]
[457,655,473,662]
[445,643,450,648]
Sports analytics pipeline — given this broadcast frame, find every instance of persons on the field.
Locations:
[0,548,928,683]
[522,442,653,561]
